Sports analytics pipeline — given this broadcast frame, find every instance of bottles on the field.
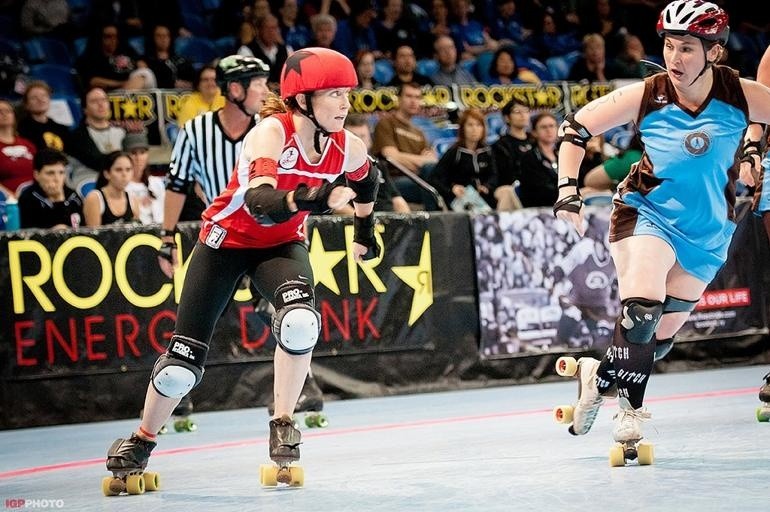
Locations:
[5,193,19,233]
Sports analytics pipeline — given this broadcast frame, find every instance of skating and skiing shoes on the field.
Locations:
[171,394,198,433]
[267,381,328,428]
[552,353,601,436]
[609,405,655,467]
[102,435,162,499]
[756,371,770,424]
[263,415,306,490]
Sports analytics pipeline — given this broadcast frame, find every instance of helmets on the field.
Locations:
[280,46,360,101]
[214,53,271,82]
[655,0,732,49]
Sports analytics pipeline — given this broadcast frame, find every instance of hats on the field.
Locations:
[121,132,150,151]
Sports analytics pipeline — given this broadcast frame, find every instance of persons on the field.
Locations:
[1,0,646,232]
[738,43,770,403]
[156,54,326,417]
[556,1,770,445]
[105,43,381,479]
